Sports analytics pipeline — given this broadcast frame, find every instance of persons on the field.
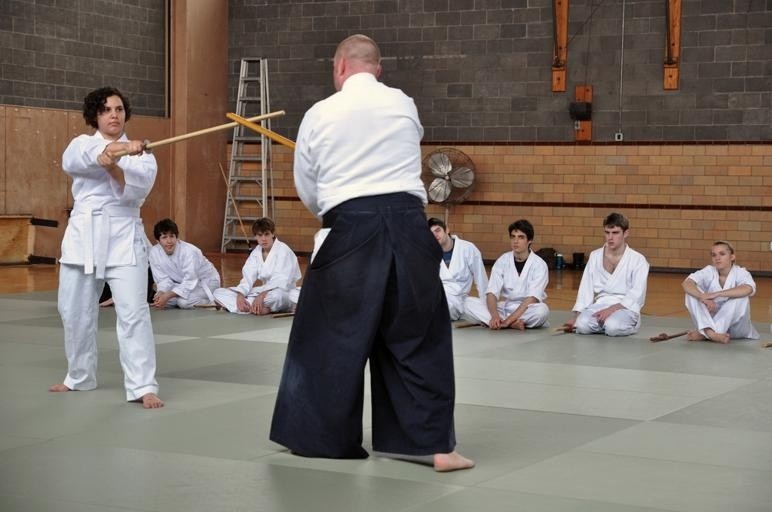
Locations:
[561,212,651,338]
[463,217,552,331]
[95,266,158,309]
[269,33,478,472]
[212,216,303,315]
[145,217,221,309]
[428,216,487,321]
[48,85,165,409]
[681,240,757,343]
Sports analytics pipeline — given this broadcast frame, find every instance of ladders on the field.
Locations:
[221,57,274,256]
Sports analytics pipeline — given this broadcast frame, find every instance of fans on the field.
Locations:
[421,147,477,229]
